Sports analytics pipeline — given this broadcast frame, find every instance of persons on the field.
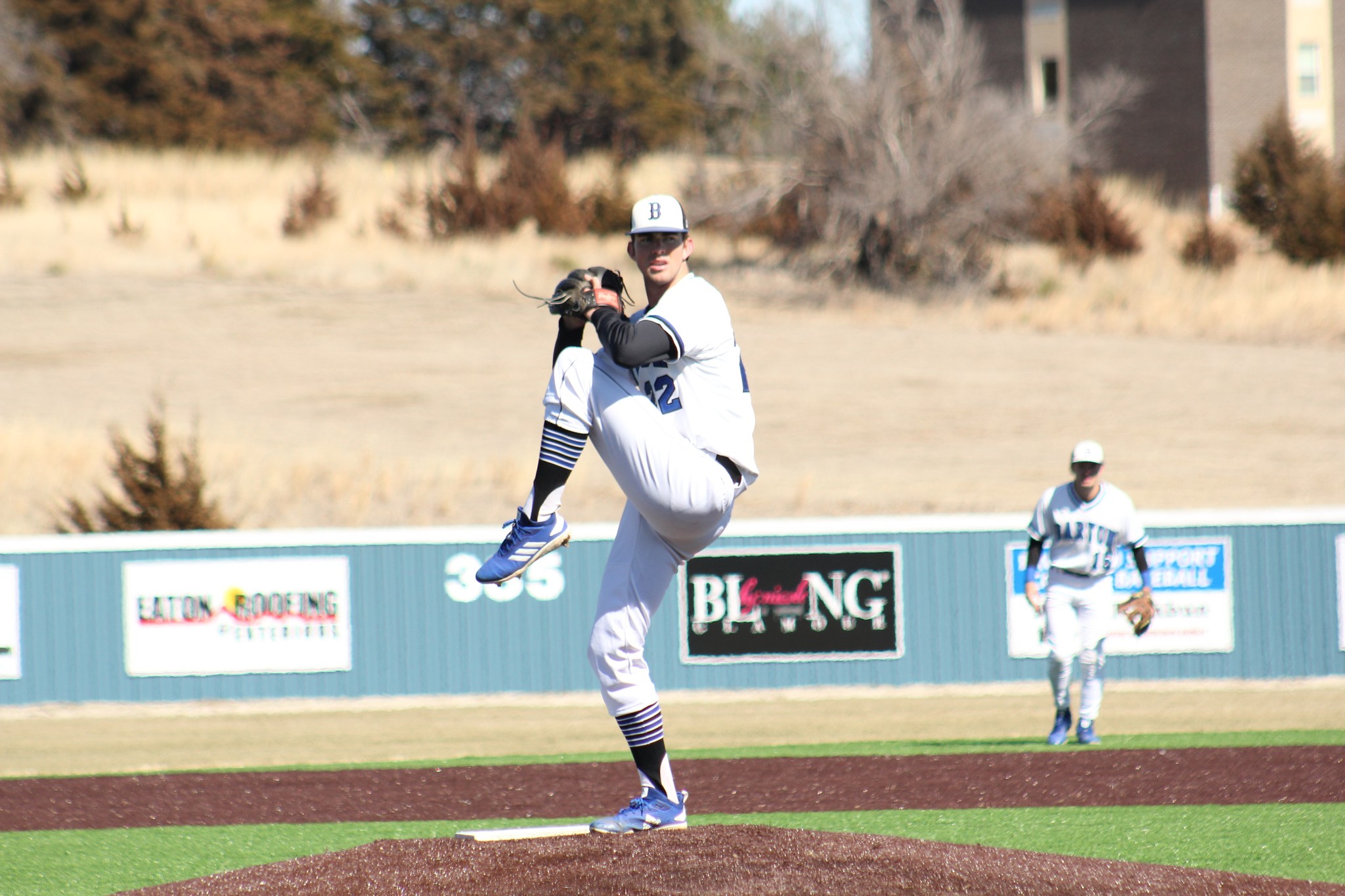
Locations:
[1025,440,1153,747]
[474,195,757,839]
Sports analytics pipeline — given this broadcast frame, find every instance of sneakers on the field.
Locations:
[1076,717,1101,745]
[476,506,572,586]
[1046,706,1072,746]
[589,786,689,834]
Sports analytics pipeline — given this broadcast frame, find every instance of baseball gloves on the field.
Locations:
[511,264,638,324]
[1116,589,1155,638]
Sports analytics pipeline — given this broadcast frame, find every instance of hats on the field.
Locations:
[1071,440,1104,465]
[625,195,688,235]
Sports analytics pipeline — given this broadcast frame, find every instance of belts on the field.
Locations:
[716,454,741,487]
[1051,565,1089,578]
